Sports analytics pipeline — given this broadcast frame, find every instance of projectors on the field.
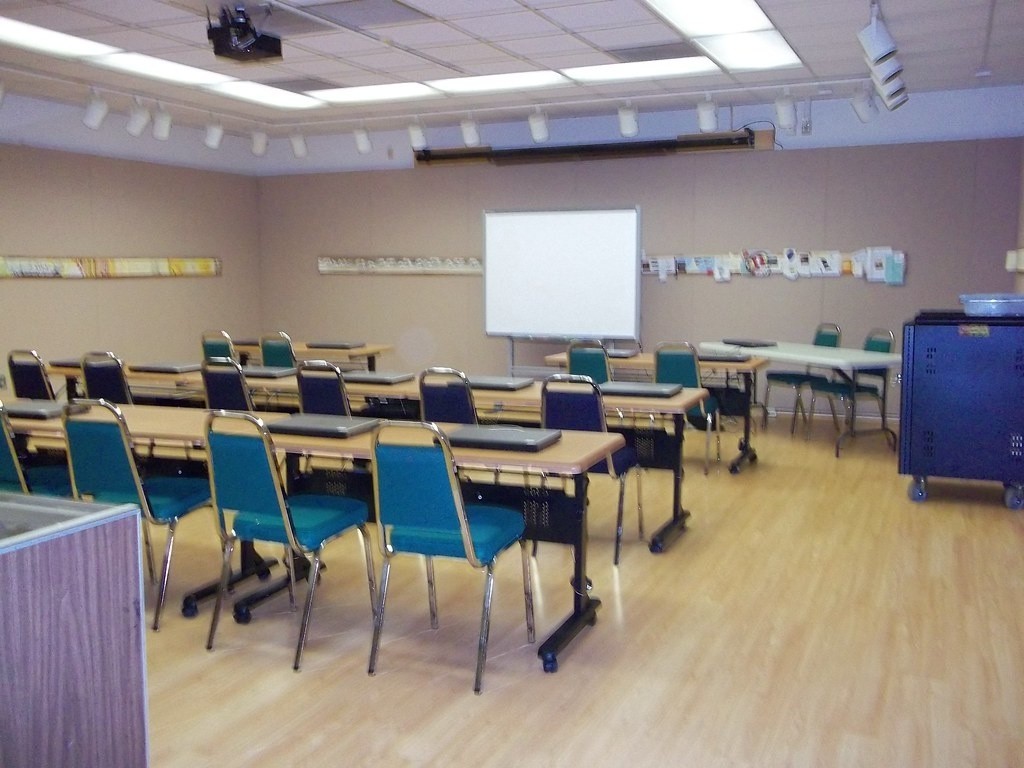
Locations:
[212,27,281,62]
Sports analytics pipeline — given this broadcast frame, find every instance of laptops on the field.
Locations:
[5,399,91,420]
[722,338,778,347]
[343,370,414,385]
[232,337,260,346]
[307,339,364,349]
[129,360,200,373]
[598,381,683,399]
[606,349,639,359]
[50,358,123,368]
[435,423,563,451]
[266,414,379,439]
[448,376,535,392]
[229,366,297,378]
[696,351,752,362]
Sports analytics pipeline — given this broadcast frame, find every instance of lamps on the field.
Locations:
[698,95,719,133]
[849,88,879,122]
[460,110,480,147]
[857,3,909,110]
[774,90,798,128]
[289,133,308,158]
[409,117,427,151]
[527,105,548,143]
[153,110,172,140]
[203,125,223,149]
[352,128,371,152]
[125,108,151,135]
[619,99,639,138]
[250,132,270,155]
[81,98,110,128]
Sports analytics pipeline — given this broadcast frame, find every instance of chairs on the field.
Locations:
[0,325,896,693]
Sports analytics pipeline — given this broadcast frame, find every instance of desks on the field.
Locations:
[0,398,626,674]
[701,338,903,460]
[229,339,393,372]
[47,370,709,553]
[543,351,768,474]
